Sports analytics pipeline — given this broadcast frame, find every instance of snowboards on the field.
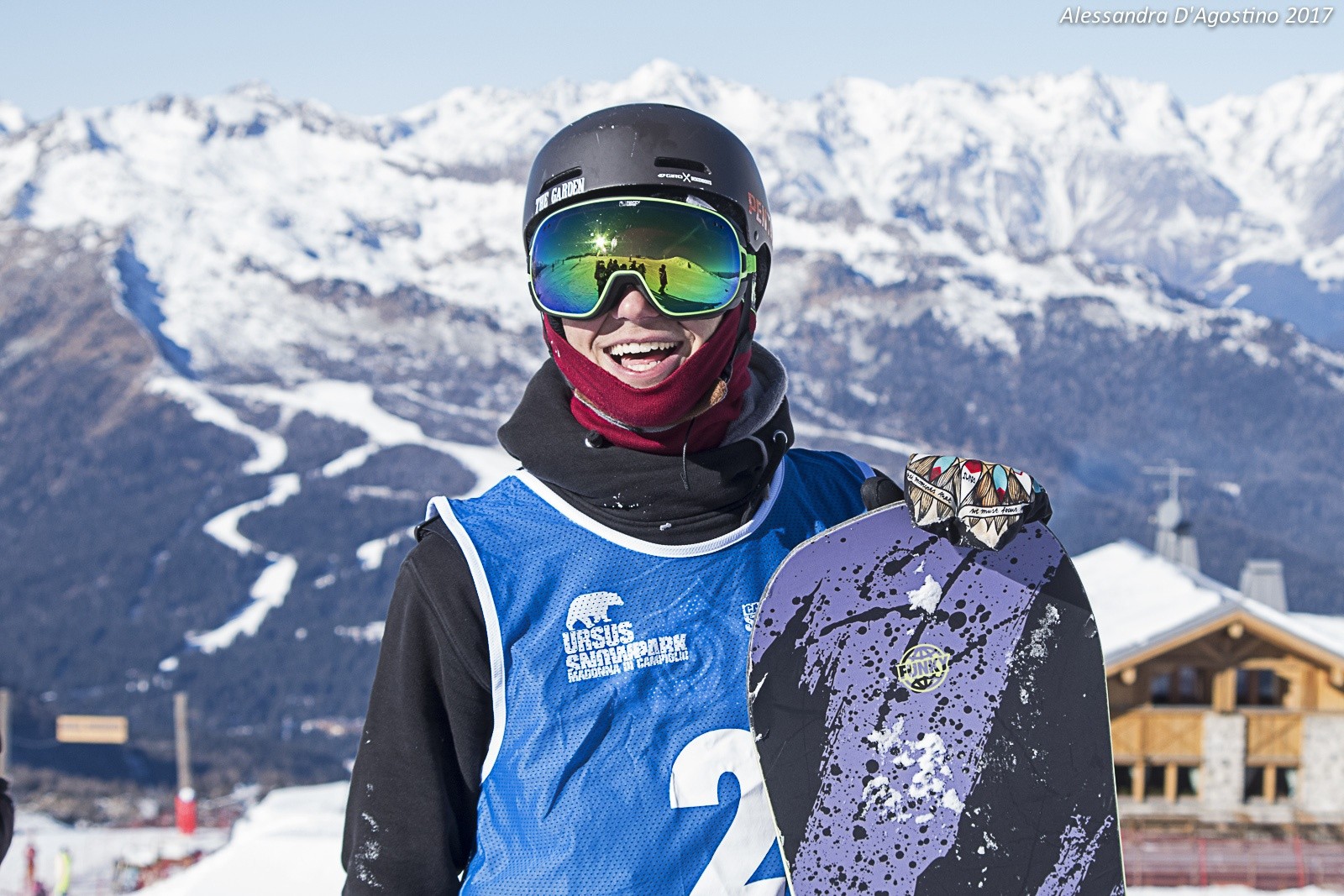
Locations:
[747,494,1129,896]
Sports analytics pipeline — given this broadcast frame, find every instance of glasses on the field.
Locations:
[528,196,757,320]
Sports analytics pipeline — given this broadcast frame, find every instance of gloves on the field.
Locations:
[859,451,1053,553]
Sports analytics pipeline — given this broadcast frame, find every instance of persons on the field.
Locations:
[0,735,15,865]
[637,263,646,280]
[629,260,638,271]
[658,264,668,294]
[342,104,1054,896]
[594,258,627,298]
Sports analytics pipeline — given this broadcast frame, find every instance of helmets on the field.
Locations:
[523,102,773,313]
